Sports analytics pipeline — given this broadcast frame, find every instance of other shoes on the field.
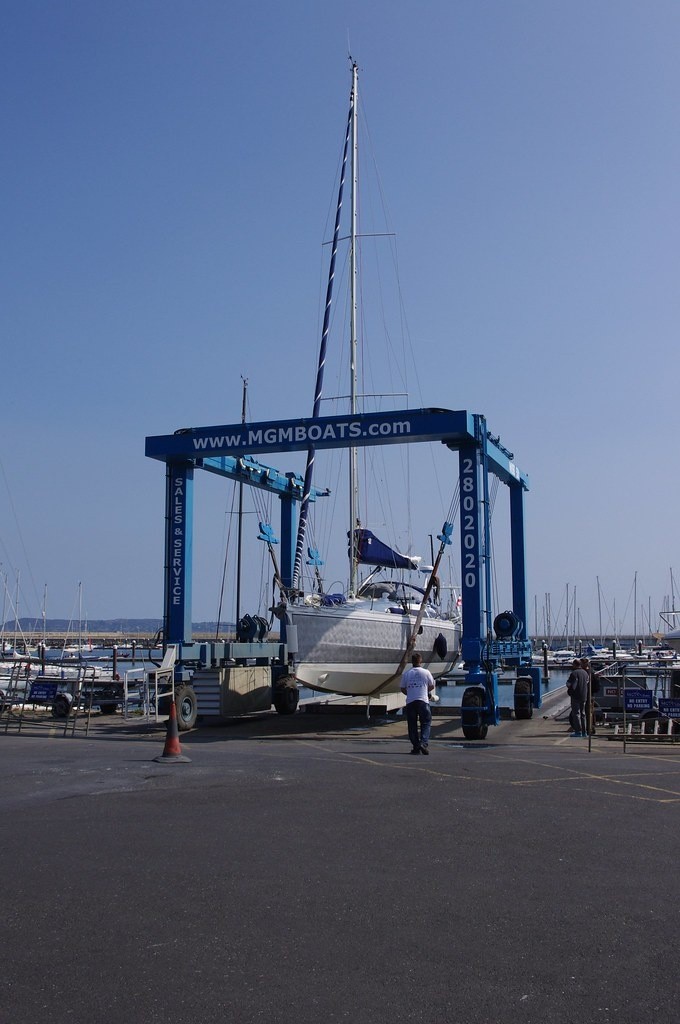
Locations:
[419,745,429,755]
[411,750,419,754]
[582,733,587,736]
[570,733,581,737]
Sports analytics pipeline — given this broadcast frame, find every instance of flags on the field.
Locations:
[457,597,462,605]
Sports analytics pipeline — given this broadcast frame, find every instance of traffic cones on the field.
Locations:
[153,702,192,763]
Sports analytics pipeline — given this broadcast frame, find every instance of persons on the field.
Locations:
[566,658,600,737]
[399,653,435,755]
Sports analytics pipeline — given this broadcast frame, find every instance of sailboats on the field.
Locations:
[0,568,163,703]
[532,568,680,668]
[279,58,462,695]
